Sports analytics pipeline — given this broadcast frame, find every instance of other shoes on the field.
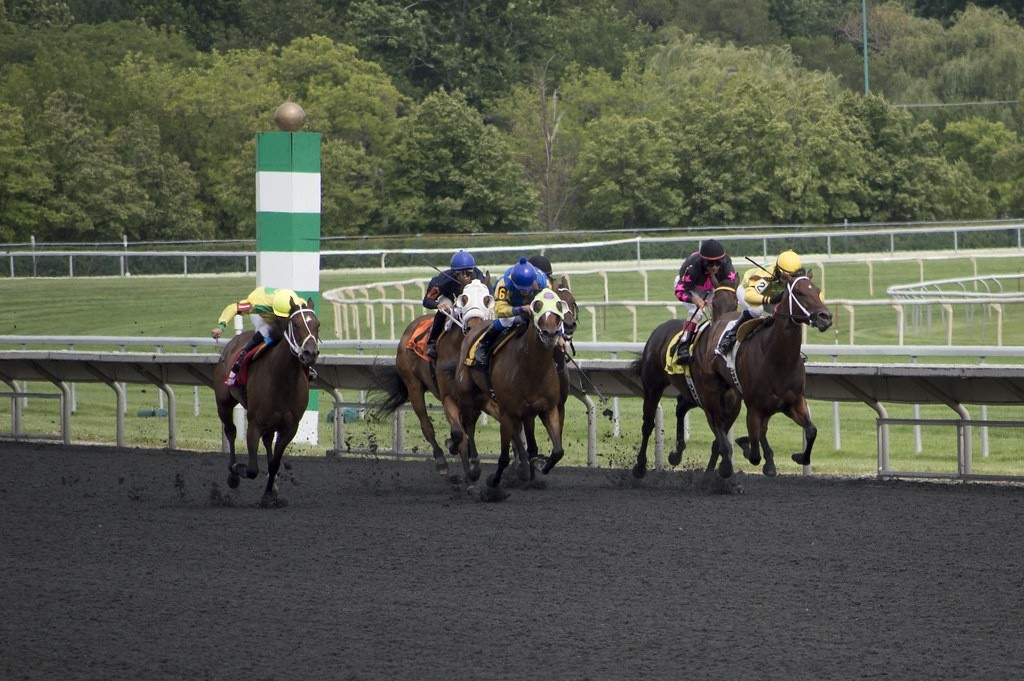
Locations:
[426,343,436,359]
[720,330,732,351]
[474,343,486,364]
[678,349,690,359]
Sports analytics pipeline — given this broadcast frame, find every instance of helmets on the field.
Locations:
[700,239,725,259]
[777,249,801,272]
[529,256,552,275]
[449,250,476,269]
[272,289,297,318]
[511,257,537,291]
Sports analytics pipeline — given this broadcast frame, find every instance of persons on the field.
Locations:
[464,255,554,372]
[423,249,496,358]
[673,239,739,365]
[211,286,307,374]
[719,249,824,355]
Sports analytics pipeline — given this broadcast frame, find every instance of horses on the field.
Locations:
[213,295,321,509]
[360,274,581,490]
[624,268,833,480]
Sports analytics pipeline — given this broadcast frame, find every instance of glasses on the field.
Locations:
[702,260,722,267]
[453,270,473,276]
